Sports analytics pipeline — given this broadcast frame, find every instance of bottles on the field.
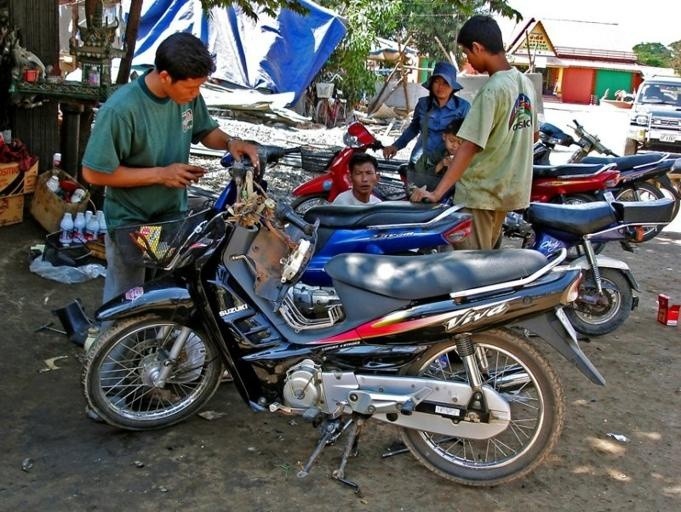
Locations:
[46,175,58,193]
[88,66,101,88]
[69,188,86,204]
[60,210,107,246]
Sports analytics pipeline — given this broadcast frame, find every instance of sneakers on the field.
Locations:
[84,384,177,424]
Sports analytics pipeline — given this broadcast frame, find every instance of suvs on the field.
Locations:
[625,76,681,156]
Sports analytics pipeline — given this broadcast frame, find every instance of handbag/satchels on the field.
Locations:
[405,164,442,195]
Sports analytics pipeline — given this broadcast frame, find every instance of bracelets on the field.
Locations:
[224,136,244,152]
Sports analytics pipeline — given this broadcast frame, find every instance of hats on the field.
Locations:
[421,62,463,92]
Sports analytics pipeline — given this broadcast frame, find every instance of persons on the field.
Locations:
[408,15,541,251]
[331,151,389,210]
[433,117,467,178]
[380,60,472,167]
[79,32,262,418]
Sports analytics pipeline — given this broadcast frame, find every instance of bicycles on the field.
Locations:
[313,70,345,127]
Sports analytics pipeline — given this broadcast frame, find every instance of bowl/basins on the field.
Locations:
[44,75,65,86]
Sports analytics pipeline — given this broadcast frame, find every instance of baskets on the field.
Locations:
[41,229,92,267]
[112,206,210,268]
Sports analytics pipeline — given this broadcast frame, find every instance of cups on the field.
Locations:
[25,68,39,84]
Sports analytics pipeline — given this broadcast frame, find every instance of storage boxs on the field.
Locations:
[0,156,92,236]
